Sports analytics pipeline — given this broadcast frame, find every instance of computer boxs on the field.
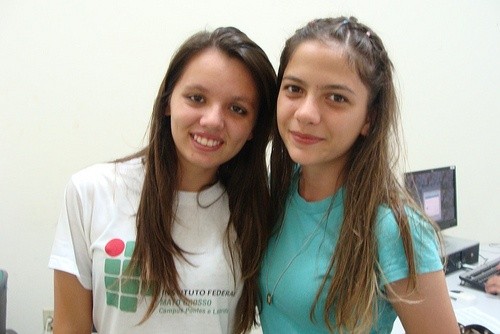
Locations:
[438,235,480,276]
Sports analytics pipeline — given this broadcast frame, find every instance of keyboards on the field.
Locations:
[458,257,500,295]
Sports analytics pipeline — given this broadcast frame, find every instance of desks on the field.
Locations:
[249,243,500,334]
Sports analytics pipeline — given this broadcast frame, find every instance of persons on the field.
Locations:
[49,27,277,334]
[257,17,460,334]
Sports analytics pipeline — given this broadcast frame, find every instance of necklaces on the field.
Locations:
[265,177,342,305]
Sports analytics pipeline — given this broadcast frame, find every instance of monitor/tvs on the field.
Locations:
[402,165,457,231]
